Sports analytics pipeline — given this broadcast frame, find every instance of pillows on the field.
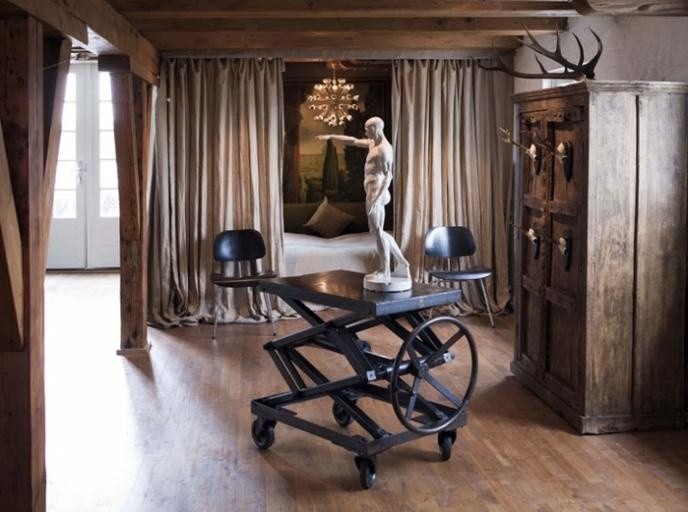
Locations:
[303,196,357,237]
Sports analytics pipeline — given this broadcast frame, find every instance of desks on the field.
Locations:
[249,270,479,485]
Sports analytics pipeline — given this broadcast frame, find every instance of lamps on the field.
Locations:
[306,61,361,127]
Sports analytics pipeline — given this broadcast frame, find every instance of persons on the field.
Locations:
[314,117,411,282]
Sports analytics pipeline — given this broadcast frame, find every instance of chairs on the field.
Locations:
[424,225,495,326]
[209,228,280,338]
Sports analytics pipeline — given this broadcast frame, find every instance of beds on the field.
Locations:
[282,200,395,311]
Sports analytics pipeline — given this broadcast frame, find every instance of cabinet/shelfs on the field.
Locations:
[509,79,687,434]
